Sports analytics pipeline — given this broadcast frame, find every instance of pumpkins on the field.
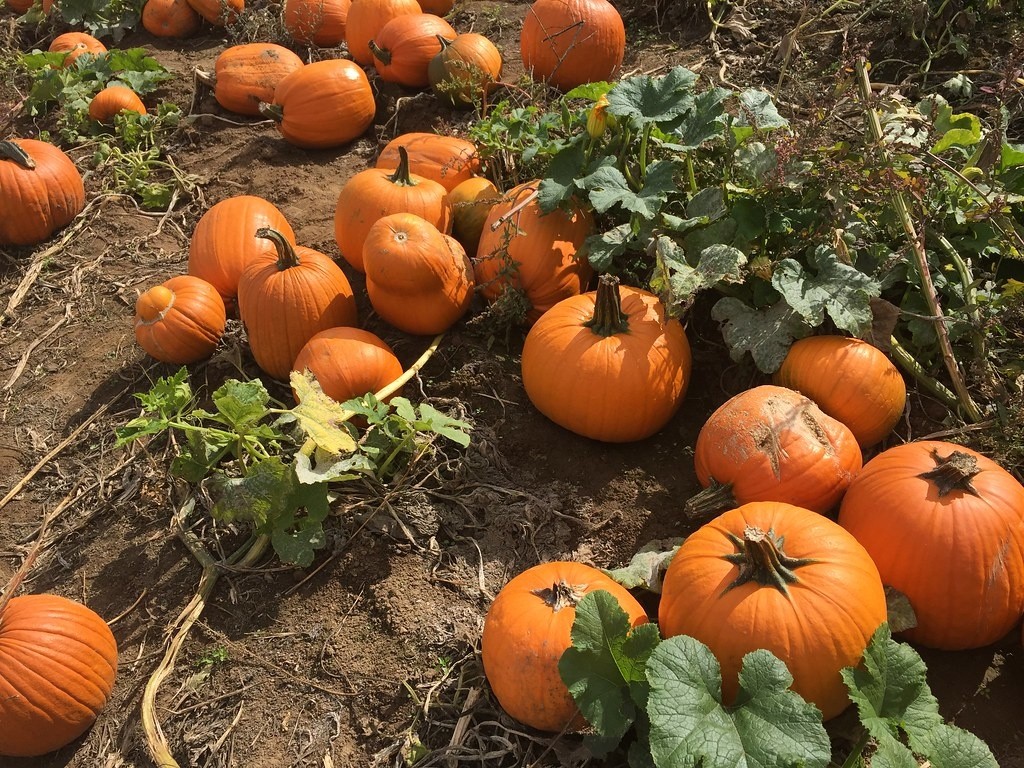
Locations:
[0,595,118,757]
[0,0,692,444]
[481,334,1024,734]
